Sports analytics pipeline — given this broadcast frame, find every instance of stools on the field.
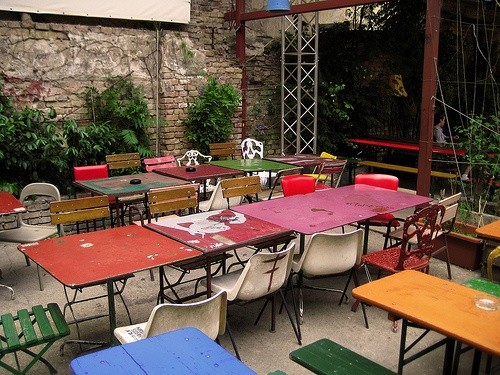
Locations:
[0,301,71,375]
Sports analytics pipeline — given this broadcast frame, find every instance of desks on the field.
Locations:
[0,138,500,375]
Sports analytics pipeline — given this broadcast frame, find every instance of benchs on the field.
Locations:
[289,337,399,375]
[357,159,460,179]
[266,369,288,375]
[459,278,500,298]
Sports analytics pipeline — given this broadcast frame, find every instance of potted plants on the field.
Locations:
[416,217,486,271]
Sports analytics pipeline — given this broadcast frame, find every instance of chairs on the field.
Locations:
[486,245,500,281]
[240,138,278,190]
[72,164,117,229]
[354,173,399,248]
[384,191,463,279]
[49,195,136,352]
[105,153,149,226]
[280,173,345,233]
[144,182,222,304]
[0,182,65,292]
[301,150,338,184]
[337,203,447,333]
[114,290,228,347]
[199,180,244,212]
[176,150,217,193]
[208,142,237,178]
[200,242,302,360]
[144,154,179,173]
[277,227,370,341]
[220,175,273,274]
